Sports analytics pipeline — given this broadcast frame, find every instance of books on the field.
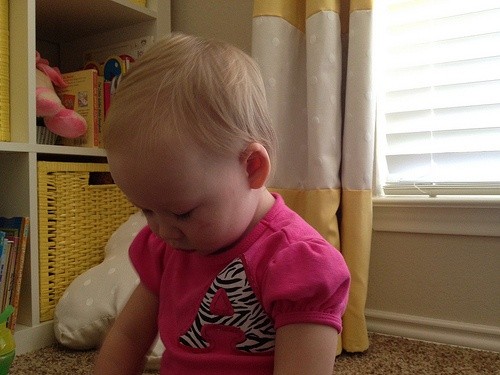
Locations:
[55,36,155,148]
[0,216,30,334]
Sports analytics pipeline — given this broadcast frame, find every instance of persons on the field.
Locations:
[94,34,350,375]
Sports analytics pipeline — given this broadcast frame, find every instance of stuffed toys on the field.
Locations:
[36,51,88,138]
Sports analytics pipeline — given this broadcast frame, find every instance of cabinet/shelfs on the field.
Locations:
[0,0,174,353]
